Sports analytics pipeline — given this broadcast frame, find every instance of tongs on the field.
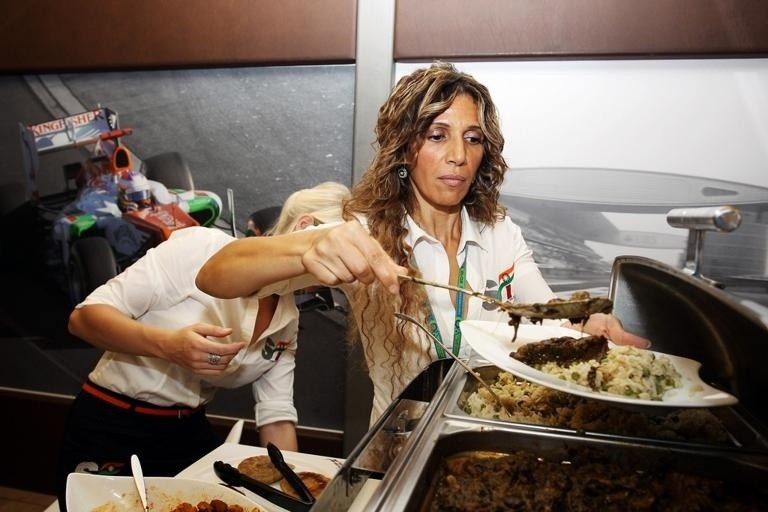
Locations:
[213,438,316,512]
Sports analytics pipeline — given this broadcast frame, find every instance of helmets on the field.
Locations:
[117,172,151,210]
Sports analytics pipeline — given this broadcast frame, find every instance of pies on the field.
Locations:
[237,454,285,484]
[280,471,332,501]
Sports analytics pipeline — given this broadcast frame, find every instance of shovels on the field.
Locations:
[393,311,529,419]
[398,275,613,318]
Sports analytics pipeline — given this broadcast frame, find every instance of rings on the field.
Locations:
[208,354,220,365]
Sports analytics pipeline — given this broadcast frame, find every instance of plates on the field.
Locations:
[458,319,738,407]
[172,441,347,512]
[65,472,268,512]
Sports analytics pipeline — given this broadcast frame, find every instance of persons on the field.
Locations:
[195,59,652,433]
[55,180,352,512]
